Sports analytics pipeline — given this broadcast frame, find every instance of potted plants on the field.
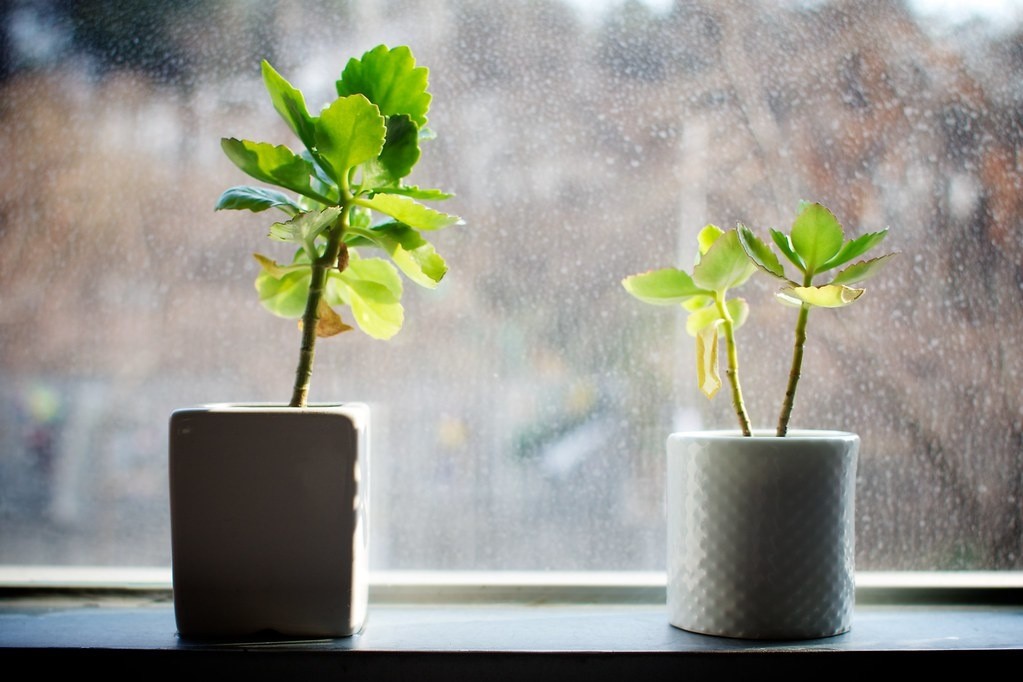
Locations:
[165,43,472,650]
[620,197,899,643]
